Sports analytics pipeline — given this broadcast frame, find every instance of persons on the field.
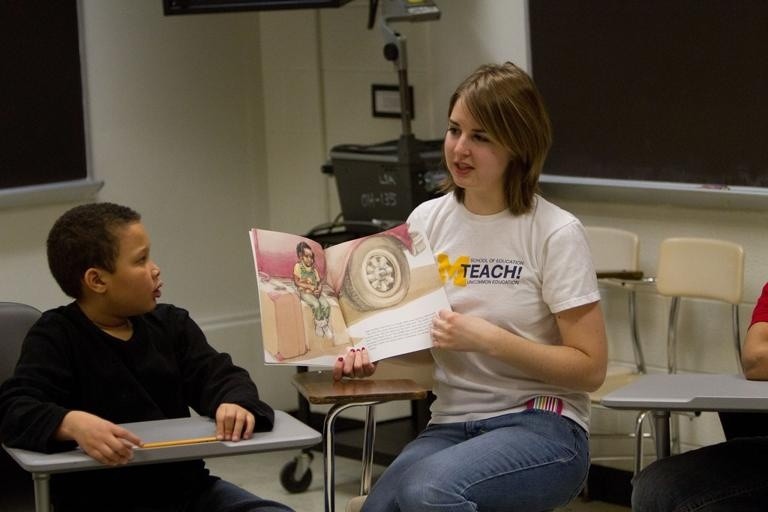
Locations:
[0,202,298,512]
[334,62,608,512]
[293,242,334,338]
[631,283,768,512]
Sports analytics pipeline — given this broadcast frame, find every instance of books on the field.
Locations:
[249,218,452,367]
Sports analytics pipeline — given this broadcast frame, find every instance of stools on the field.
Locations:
[291,372,429,511]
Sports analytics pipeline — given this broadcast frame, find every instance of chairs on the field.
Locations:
[654,235,745,375]
[586,225,641,488]
[0,295,44,383]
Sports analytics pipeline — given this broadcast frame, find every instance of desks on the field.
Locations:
[7,409,323,511]
[598,373,766,510]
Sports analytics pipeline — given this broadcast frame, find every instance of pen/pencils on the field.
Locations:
[699,183,729,190]
[140,437,219,448]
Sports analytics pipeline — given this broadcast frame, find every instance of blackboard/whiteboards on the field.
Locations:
[525,1,767,202]
[3,2,104,205]
